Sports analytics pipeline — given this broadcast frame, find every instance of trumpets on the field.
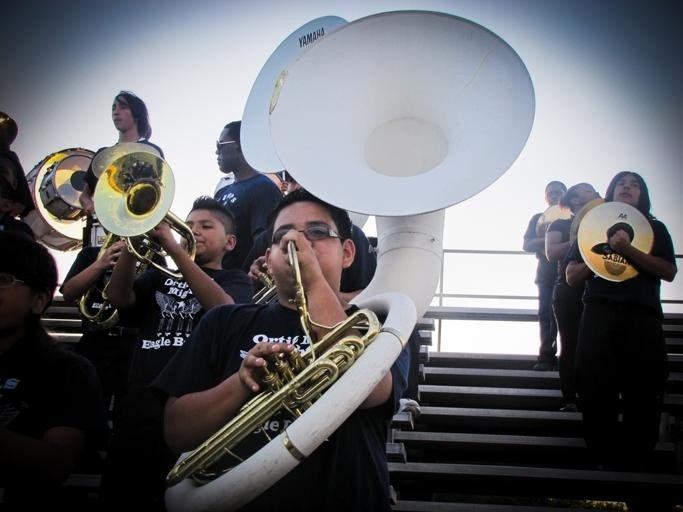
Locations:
[79,141,163,327]
[94,152,196,278]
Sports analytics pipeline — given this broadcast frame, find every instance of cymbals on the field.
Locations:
[537,196,654,283]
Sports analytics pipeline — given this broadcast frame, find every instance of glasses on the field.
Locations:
[269,226,343,244]
[0,274,24,288]
[216,140,235,149]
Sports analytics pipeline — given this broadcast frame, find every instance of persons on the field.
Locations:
[564,172,677,474]
[213,121,283,274]
[523,180,568,371]
[1,112,38,219]
[545,184,604,412]
[107,195,252,426]
[241,182,378,300]
[59,91,164,426]
[0,233,110,510]
[152,189,410,510]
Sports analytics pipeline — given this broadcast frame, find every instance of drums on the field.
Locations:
[20,148,97,252]
[40,154,94,223]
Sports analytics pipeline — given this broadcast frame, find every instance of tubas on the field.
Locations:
[164,10,535,512]
[241,15,368,309]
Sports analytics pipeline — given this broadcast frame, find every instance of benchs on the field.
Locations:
[645,311,681,455]
[38,294,91,350]
[378,314,435,500]
